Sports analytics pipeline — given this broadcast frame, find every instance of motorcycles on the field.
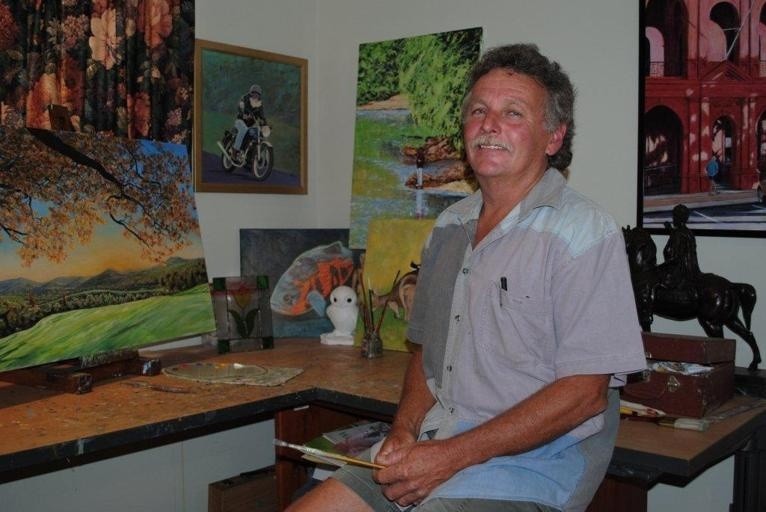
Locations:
[215,110,275,182]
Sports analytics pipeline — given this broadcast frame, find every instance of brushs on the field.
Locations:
[619,413,708,431]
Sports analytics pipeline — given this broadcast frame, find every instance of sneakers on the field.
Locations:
[231,146,236,159]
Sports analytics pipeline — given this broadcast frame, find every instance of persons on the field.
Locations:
[230,84,266,162]
[658,198,700,292]
[287,43,654,511]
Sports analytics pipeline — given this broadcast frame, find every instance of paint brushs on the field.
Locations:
[272,438,387,469]
[360,269,400,333]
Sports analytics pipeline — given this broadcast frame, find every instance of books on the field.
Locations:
[297,419,391,484]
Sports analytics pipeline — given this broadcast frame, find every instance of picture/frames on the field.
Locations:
[194,38,308,195]
[637,0,766,238]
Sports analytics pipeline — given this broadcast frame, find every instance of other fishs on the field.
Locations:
[268,241,355,319]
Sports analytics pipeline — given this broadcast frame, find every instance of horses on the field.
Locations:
[621,224,763,373]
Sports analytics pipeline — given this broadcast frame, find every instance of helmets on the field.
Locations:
[250,84,262,96]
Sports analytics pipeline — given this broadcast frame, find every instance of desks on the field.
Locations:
[0,337,766,512]
